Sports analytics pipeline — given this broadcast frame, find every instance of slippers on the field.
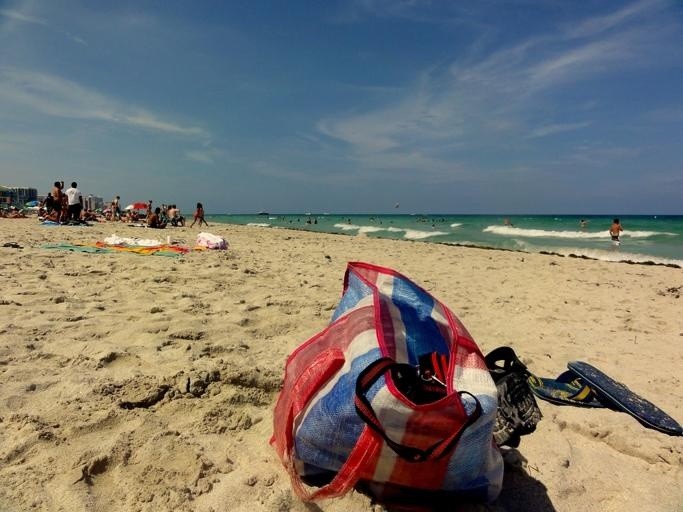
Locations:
[568,361,683,435]
[524,371,619,408]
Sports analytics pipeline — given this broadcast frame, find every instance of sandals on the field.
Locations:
[485,347,543,448]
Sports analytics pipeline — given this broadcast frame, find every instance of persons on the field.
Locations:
[1,180,184,228]
[189,203,208,228]
[607,216,624,246]
[577,218,587,229]
[502,215,513,228]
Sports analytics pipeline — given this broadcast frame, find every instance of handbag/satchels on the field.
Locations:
[269,261,505,511]
